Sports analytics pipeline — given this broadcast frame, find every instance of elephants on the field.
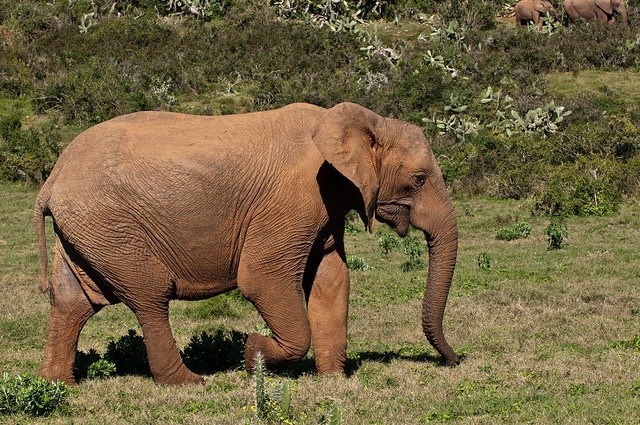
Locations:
[514,0,563,29]
[563,0,632,31]
[33,101,462,383]
